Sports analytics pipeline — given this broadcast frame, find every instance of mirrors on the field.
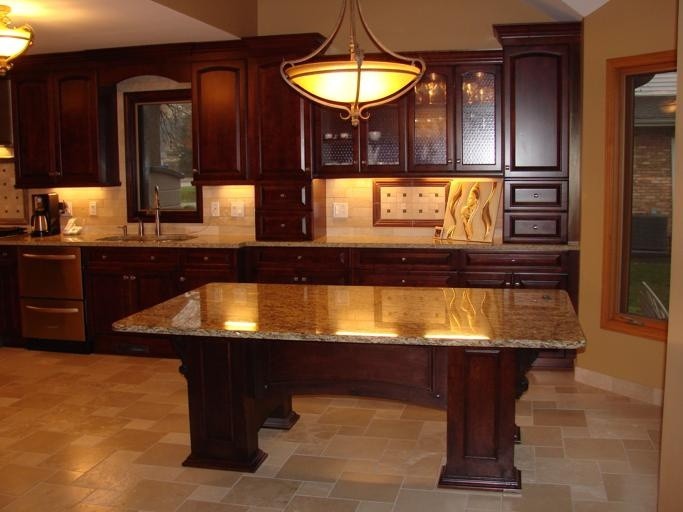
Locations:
[122,89,205,223]
[598,49,678,340]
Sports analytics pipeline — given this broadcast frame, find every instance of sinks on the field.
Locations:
[94,232,196,244]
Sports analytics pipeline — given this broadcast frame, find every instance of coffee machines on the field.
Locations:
[31,193,59,236]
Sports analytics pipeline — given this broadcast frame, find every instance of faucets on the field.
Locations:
[152,182,164,236]
[136,213,145,237]
[116,224,128,236]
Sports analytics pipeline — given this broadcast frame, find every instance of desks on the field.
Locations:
[114,280,588,493]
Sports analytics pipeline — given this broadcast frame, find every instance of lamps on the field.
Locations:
[279,0,427,127]
[0,0,37,76]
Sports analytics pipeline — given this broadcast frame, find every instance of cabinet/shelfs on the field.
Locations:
[172,242,242,363]
[84,250,178,363]
[2,245,86,354]
[492,16,583,244]
[245,248,352,284]
[355,247,460,284]
[402,48,502,185]
[8,50,102,194]
[459,250,580,371]
[191,33,327,242]
[311,55,409,178]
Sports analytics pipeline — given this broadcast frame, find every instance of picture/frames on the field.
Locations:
[374,180,451,228]
[0,157,31,232]
[439,179,503,246]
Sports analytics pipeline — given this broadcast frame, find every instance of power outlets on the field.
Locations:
[211,202,221,216]
[333,203,349,218]
[229,201,245,217]
[61,202,72,216]
